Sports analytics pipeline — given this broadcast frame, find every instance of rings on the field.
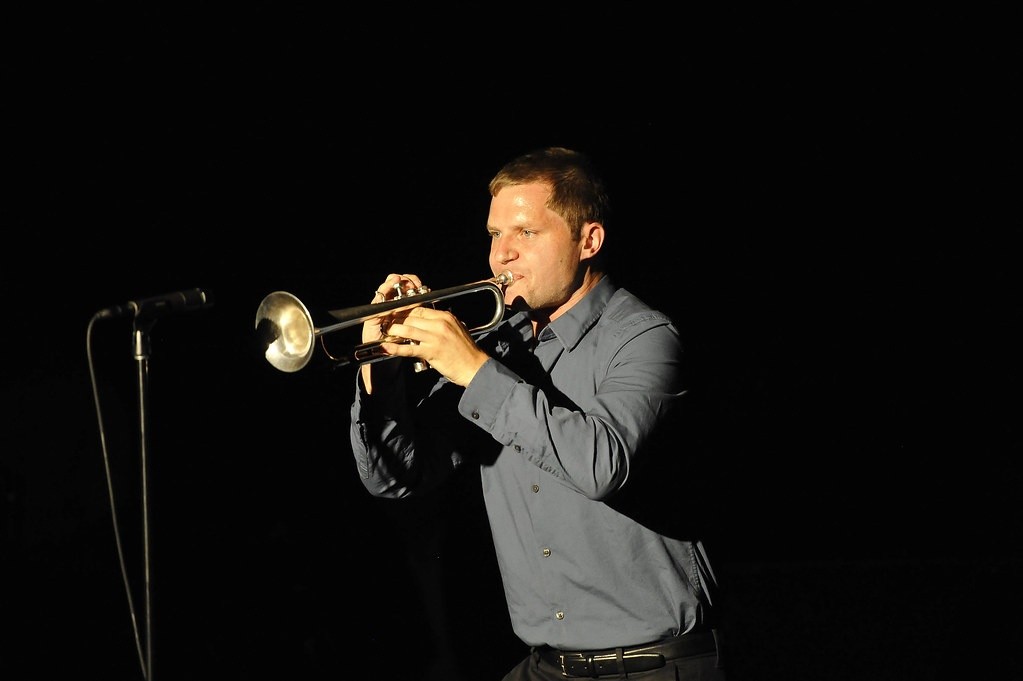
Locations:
[375,291,385,300]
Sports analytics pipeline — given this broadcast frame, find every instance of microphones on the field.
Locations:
[99,286,215,319]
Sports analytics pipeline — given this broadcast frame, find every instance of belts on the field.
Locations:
[535,631,723,678]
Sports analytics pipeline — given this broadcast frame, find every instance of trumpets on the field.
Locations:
[253,268,517,376]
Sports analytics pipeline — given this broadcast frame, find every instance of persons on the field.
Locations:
[348,145,739,681]
